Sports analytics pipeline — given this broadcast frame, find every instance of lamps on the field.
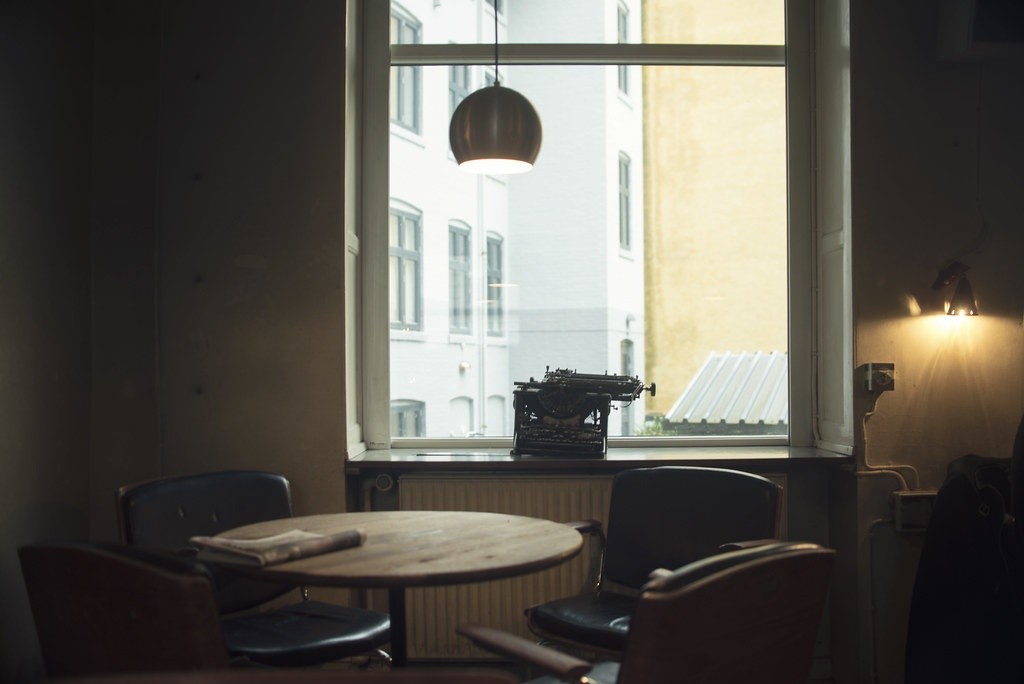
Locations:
[448,0,543,173]
[940,268,978,316]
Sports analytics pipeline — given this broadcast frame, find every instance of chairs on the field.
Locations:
[17,467,395,677]
[451,538,837,683]
[523,466,784,680]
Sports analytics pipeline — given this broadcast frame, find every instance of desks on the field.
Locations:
[198,512,581,668]
